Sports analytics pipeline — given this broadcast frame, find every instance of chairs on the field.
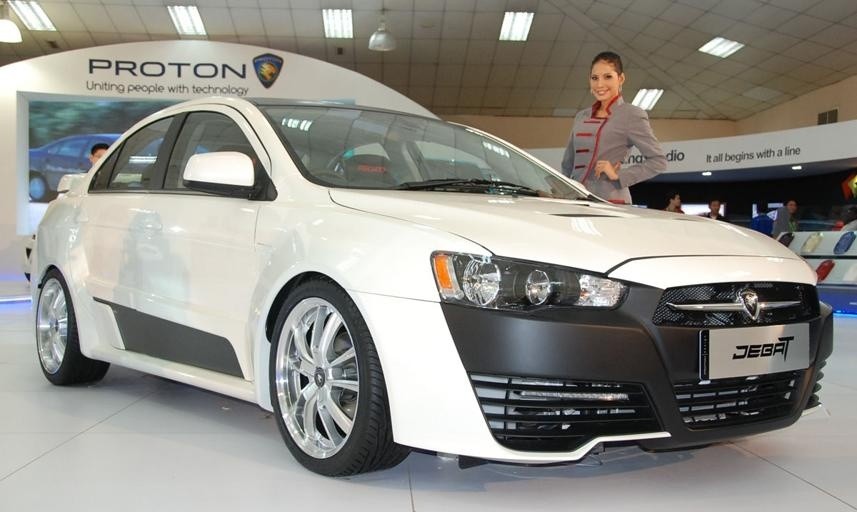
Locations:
[344,154,396,185]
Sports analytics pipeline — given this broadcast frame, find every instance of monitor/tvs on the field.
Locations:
[752,203,784,221]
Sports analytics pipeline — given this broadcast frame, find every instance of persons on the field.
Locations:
[771,199,800,240]
[85,142,110,167]
[661,191,684,214]
[747,199,775,239]
[559,51,667,208]
[696,198,727,222]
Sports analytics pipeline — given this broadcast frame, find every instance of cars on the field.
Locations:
[30,132,205,200]
[28,97,833,477]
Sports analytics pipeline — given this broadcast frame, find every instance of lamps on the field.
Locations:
[368,13,397,53]
